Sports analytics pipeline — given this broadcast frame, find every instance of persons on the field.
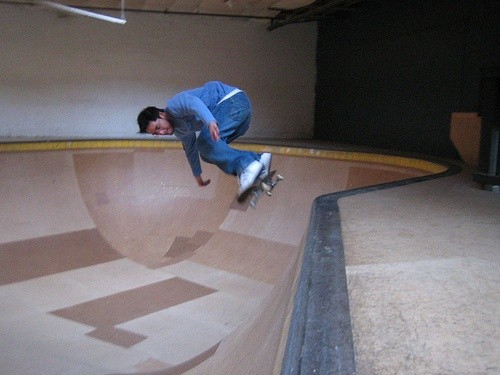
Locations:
[136,81,272,201]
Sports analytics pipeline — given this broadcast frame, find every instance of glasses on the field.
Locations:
[154,120,160,140]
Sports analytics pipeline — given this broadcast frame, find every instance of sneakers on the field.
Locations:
[259,152,273,180]
[236,159,265,201]
